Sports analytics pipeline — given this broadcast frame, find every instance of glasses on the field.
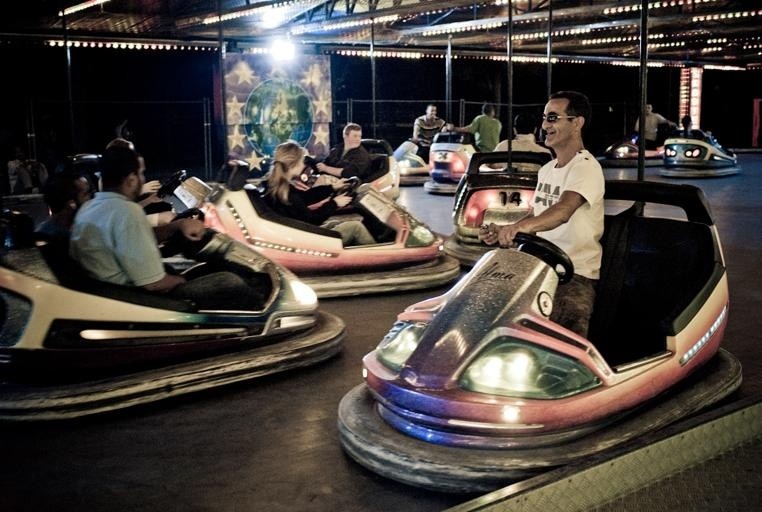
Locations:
[543,116,576,121]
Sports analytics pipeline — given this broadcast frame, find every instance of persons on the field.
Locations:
[265,140,377,247]
[6,137,222,239]
[412,104,451,145]
[445,102,503,152]
[678,115,712,142]
[66,137,266,310]
[488,110,553,172]
[634,101,676,147]
[476,90,607,342]
